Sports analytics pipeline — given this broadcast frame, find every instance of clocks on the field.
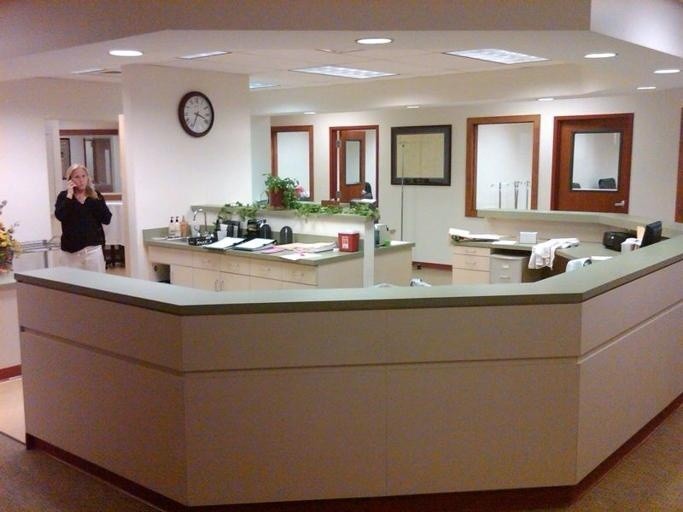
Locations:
[178,91,215,137]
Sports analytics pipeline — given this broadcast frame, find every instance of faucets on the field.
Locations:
[41,233,62,244]
[193,208,207,233]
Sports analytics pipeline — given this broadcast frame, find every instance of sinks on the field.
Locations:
[34,245,62,250]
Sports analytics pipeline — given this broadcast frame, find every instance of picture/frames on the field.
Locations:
[391,124,451,186]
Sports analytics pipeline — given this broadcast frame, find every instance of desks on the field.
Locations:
[448,234,622,284]
[144,225,416,292]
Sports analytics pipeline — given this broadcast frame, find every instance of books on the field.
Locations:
[201,236,336,254]
[620,237,641,255]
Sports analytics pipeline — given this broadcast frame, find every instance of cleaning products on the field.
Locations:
[180,216,187,238]
[168,216,175,239]
[175,216,180,238]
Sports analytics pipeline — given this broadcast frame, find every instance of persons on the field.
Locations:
[55,164,112,272]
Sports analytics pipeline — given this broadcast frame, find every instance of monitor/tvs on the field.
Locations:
[639,220,662,248]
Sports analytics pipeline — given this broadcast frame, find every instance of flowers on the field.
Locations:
[264,172,304,210]
[1,198,23,274]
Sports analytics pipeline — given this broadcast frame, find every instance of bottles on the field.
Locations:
[169,216,187,238]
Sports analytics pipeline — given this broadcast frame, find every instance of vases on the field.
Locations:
[269,187,284,206]
[1,248,14,270]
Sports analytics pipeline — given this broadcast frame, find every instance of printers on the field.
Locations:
[374,223,390,246]
